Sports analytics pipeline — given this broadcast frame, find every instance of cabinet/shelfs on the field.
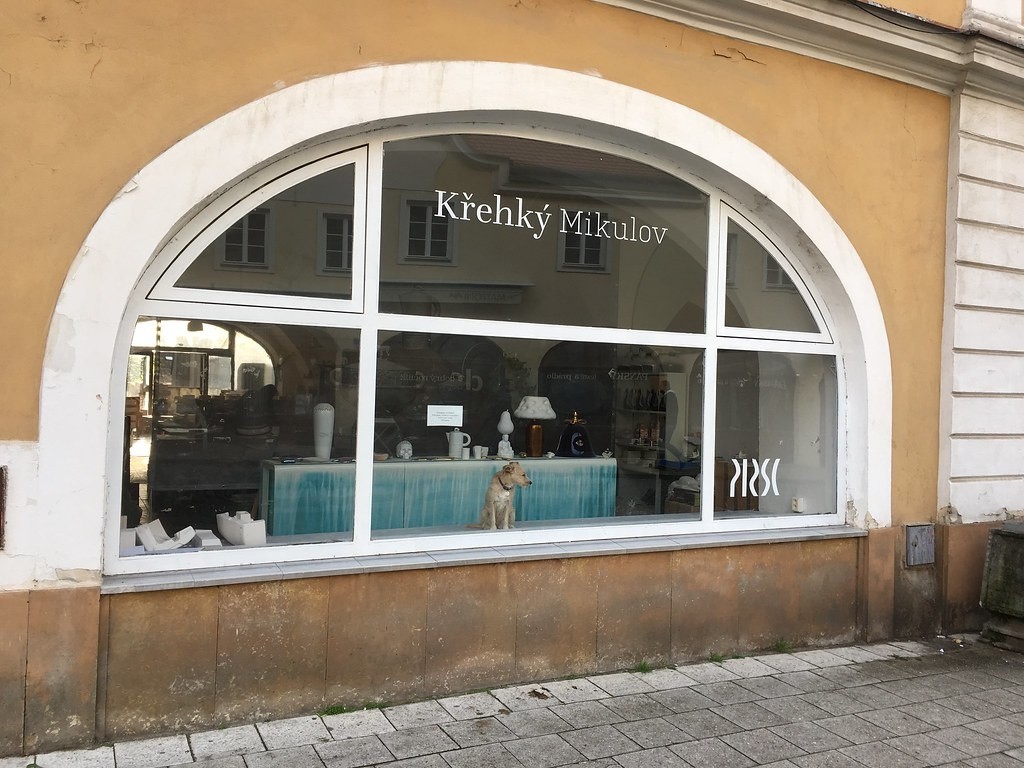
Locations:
[612,373,689,516]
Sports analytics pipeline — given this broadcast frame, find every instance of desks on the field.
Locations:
[260,456,617,537]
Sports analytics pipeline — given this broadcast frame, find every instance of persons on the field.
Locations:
[658,380,686,469]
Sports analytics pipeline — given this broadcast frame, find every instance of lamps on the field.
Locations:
[513,395,556,457]
[187,322,203,331]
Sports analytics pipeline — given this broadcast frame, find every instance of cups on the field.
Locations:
[473,446,482,459]
[462,448,470,459]
[187,414,196,427]
[481,447,489,457]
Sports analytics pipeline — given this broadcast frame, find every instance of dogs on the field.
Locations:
[467,461,532,530]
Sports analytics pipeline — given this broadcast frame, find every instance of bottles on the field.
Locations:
[623,387,666,411]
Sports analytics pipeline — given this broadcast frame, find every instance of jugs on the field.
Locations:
[446,428,471,458]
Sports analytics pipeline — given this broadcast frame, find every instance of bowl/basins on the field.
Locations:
[375,454,389,460]
